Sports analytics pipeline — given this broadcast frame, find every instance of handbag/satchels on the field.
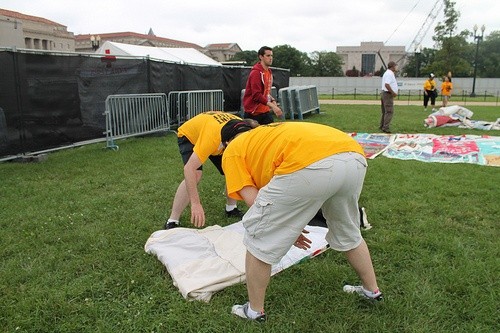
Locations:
[432,89,437,97]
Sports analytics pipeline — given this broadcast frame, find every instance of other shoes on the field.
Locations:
[164,220,180,230]
[343,284,384,304]
[231,302,265,322]
[225,207,244,217]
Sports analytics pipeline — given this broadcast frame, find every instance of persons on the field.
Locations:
[243,46,282,125]
[424,73,438,111]
[440,74,453,107]
[380,61,400,134]
[165,110,260,231]
[221,119,384,321]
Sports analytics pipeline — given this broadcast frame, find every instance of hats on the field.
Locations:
[388,62,397,68]
[429,73,435,77]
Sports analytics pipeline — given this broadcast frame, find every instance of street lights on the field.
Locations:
[470,24,486,97]
[90,35,101,51]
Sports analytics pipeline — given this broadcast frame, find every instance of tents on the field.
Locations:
[90,40,223,66]
